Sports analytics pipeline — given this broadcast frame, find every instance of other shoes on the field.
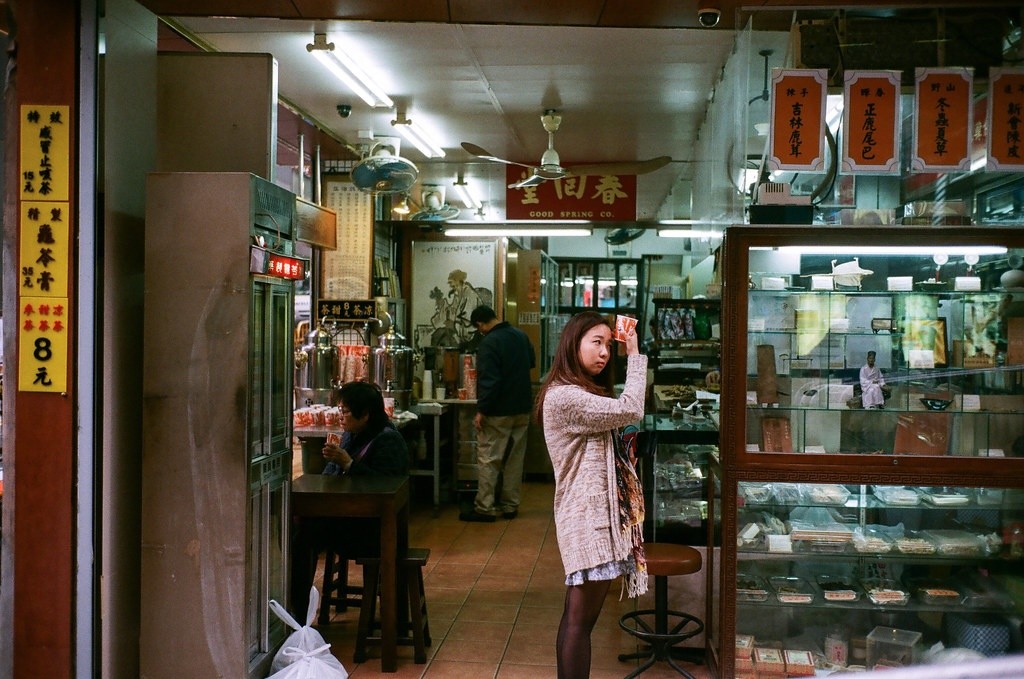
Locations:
[502,509,517,519]
[459,510,496,522]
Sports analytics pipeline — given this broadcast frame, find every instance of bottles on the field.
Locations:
[417,431,427,460]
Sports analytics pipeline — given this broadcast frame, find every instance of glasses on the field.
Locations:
[338,405,352,416]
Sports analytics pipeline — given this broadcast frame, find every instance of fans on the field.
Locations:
[462,111,673,188]
[604,228,646,246]
[351,138,420,197]
[407,185,461,221]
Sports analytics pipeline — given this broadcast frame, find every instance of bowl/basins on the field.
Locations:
[919,398,953,410]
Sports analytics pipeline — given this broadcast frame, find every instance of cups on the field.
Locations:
[326,433,342,449]
[335,345,371,384]
[422,370,446,400]
[293,398,394,428]
[614,315,639,343]
[458,355,476,400]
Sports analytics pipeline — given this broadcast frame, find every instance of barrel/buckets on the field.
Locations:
[424,346,459,399]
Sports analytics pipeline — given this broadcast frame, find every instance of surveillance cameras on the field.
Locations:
[336,104,352,118]
[698,8,720,27]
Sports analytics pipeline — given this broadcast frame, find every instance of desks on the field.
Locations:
[291,475,409,672]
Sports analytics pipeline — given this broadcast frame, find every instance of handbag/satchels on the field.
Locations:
[265,586,349,679]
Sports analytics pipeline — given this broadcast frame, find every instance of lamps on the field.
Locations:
[452,176,482,209]
[306,35,395,109]
[394,197,410,214]
[441,222,593,237]
[656,225,723,238]
[391,113,447,159]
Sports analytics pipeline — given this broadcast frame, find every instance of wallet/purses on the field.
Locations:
[623,430,657,460]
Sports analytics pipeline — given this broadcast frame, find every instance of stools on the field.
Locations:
[618,543,705,679]
[317,546,432,665]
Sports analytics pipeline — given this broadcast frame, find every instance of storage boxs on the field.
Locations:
[946,613,1011,657]
[867,626,923,668]
[734,634,817,679]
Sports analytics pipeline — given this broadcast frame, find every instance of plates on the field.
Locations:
[915,282,947,291]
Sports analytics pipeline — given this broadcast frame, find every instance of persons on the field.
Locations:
[860,351,886,409]
[459,305,536,521]
[292,382,408,626]
[535,311,648,679]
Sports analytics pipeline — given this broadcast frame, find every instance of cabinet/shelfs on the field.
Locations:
[408,403,501,519]
[705,225,1024,679]
[635,298,722,548]
[517,249,559,389]
